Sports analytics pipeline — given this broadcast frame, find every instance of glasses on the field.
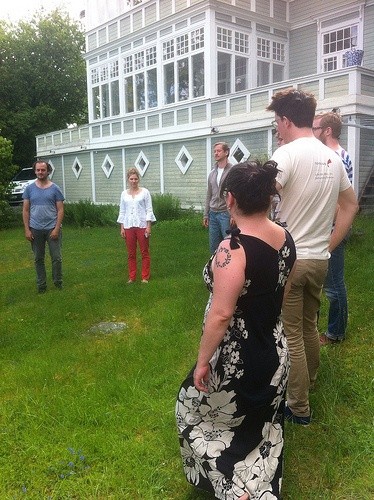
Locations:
[271,119,283,128]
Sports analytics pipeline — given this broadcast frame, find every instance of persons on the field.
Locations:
[174,89,359,500]
[22,160,65,293]
[117,167,157,284]
[235,76,242,91]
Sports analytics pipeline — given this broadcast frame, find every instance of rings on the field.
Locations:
[150,234,151,236]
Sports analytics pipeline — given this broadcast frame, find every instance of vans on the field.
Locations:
[5,168,38,206]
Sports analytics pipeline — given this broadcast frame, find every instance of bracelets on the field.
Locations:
[329,248,333,253]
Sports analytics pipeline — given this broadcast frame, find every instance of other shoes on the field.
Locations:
[37,285,46,293]
[318,334,341,345]
[126,279,136,284]
[56,284,62,290]
[141,279,148,284]
[283,403,311,424]
